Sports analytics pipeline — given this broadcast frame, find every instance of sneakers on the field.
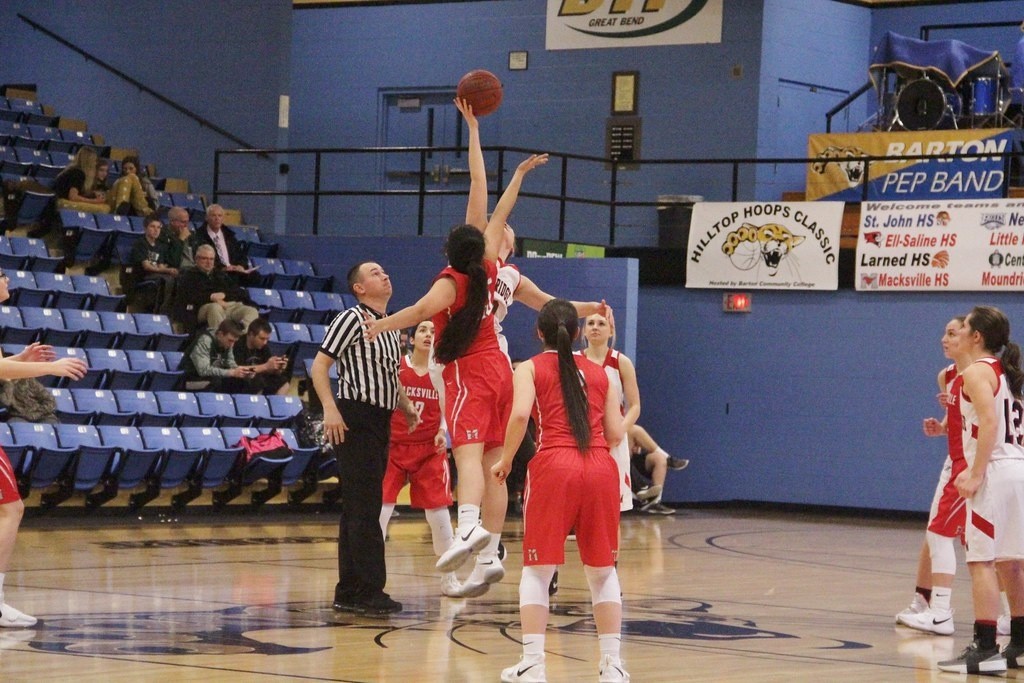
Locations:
[435,519,491,573]
[0,604,37,627]
[598,654,630,683]
[995,613,1011,635]
[894,593,929,622]
[501,654,546,683]
[937,644,1007,672]
[459,553,505,598]
[648,502,675,514]
[640,495,661,510]
[440,595,466,619]
[898,611,954,635]
[637,486,661,499]
[1000,643,1024,666]
[332,589,402,614]
[440,575,463,596]
[667,455,689,470]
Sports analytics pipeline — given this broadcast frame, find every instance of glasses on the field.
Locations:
[0,273,6,278]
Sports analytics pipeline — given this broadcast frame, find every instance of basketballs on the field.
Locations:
[456,69,504,117]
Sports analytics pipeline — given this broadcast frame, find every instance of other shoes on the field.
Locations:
[549,571,559,595]
[497,541,507,561]
[567,529,576,541]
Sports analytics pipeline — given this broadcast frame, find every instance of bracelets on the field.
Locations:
[439,416,448,433]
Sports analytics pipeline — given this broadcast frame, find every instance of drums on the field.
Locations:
[969,77,1004,116]
[895,79,962,131]
[895,64,920,80]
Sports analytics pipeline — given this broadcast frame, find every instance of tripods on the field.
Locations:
[978,59,1024,130]
[857,68,892,132]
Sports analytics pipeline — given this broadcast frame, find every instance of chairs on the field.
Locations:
[0,86,420,506]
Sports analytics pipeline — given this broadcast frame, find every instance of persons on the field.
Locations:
[309,260,424,613]
[449,89,613,597]
[935,304,1024,677]
[295,356,690,515]
[572,312,642,597]
[897,315,1011,634]
[895,392,947,625]
[361,151,550,597]
[0,263,88,631]
[379,319,454,555]
[490,297,632,683]
[55,143,297,396]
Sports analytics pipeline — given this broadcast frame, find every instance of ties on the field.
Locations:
[214,235,225,265]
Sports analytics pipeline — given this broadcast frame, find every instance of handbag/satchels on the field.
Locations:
[230,427,294,465]
